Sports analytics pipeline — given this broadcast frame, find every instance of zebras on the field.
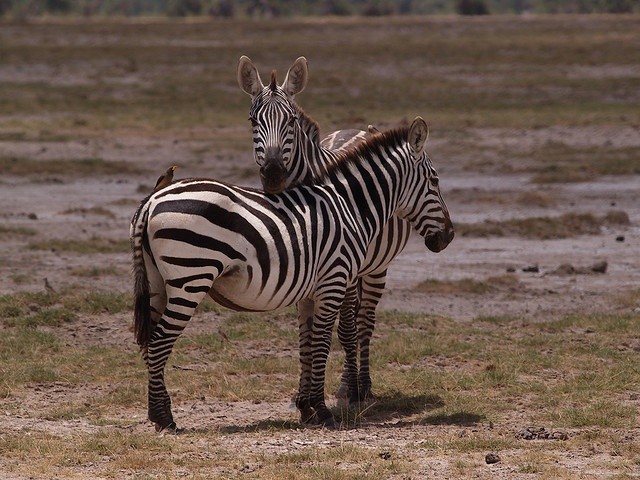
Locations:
[235,52,416,405]
[128,114,455,433]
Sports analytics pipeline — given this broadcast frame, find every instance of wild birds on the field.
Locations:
[154,165,178,191]
